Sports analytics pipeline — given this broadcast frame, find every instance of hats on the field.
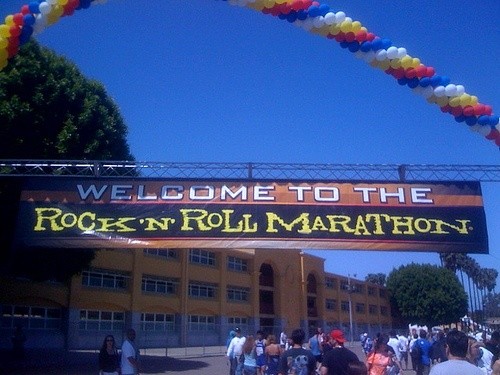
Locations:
[330,330,346,342]
[233,327,241,332]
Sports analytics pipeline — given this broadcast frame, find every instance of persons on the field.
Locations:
[360,327,500,375]
[225,327,334,375]
[429,330,483,375]
[120,328,144,375]
[277,329,316,375]
[321,329,358,375]
[98,334,120,375]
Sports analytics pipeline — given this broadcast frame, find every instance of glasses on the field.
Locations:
[107,339,113,342]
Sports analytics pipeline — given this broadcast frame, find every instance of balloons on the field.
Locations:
[0,0,107,71]
[227,0,500,151]
